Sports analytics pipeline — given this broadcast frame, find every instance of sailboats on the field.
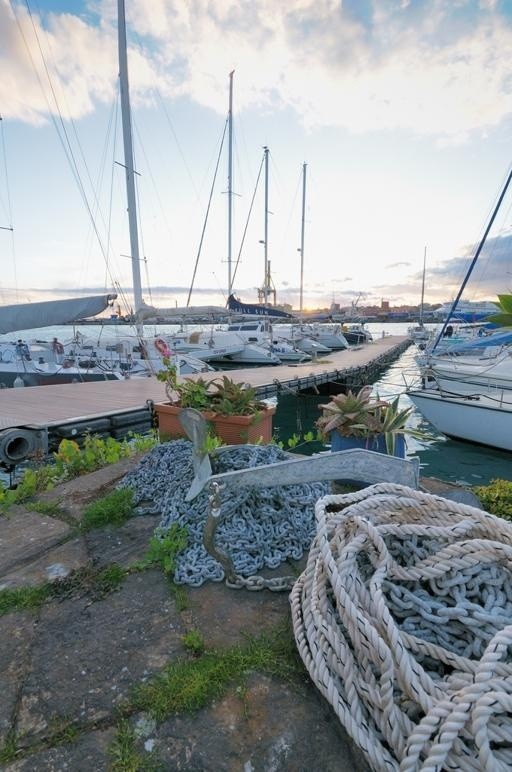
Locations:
[401,0,512,452]
[0,0,374,378]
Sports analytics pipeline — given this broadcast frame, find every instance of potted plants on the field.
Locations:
[315,385,441,488]
[153,358,277,447]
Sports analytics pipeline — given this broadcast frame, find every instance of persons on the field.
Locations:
[53,337,65,354]
[17,339,31,361]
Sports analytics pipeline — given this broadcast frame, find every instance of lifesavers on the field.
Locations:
[155,338,169,353]
